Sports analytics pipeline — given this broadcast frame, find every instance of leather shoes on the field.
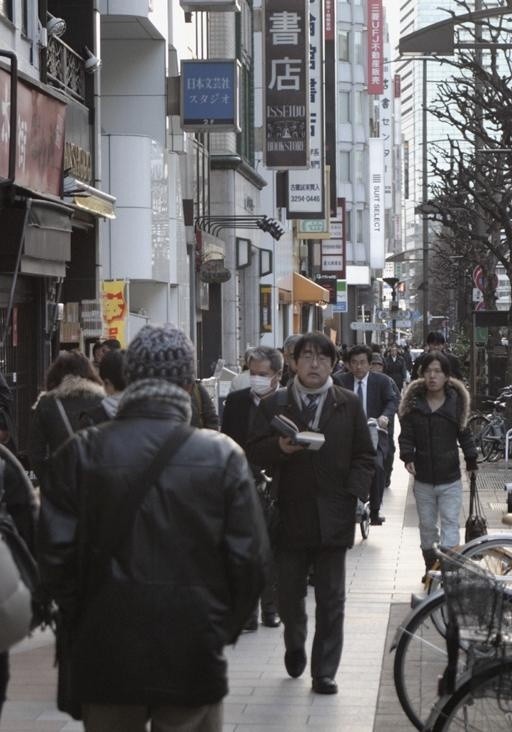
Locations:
[313,677,338,694]
[263,613,281,626]
[284,649,307,677]
[244,618,258,629]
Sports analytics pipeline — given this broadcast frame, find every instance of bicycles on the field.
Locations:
[389,570,512,730]
[464,384,512,464]
[422,542,512,732]
[414,534,512,645]
[359,420,387,542]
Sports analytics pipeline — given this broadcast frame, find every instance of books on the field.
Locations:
[270,411,325,452]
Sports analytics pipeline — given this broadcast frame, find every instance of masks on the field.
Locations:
[250,374,279,395]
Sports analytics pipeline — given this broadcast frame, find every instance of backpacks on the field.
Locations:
[1,504,50,652]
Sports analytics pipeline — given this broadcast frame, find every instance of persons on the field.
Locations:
[1,375,53,715]
[102,340,120,353]
[93,343,105,363]
[329,333,462,380]
[372,350,386,378]
[83,353,131,427]
[333,346,400,525]
[220,346,285,632]
[280,334,307,387]
[190,377,218,433]
[28,352,105,491]
[245,332,378,695]
[47,325,272,732]
[398,352,487,584]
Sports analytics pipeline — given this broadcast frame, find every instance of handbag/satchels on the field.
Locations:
[465,475,487,561]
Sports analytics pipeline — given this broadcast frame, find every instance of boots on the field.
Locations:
[421,548,438,584]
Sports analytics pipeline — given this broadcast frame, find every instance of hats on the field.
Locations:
[123,325,197,387]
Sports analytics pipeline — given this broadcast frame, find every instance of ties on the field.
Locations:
[303,394,322,427]
[357,381,363,407]
